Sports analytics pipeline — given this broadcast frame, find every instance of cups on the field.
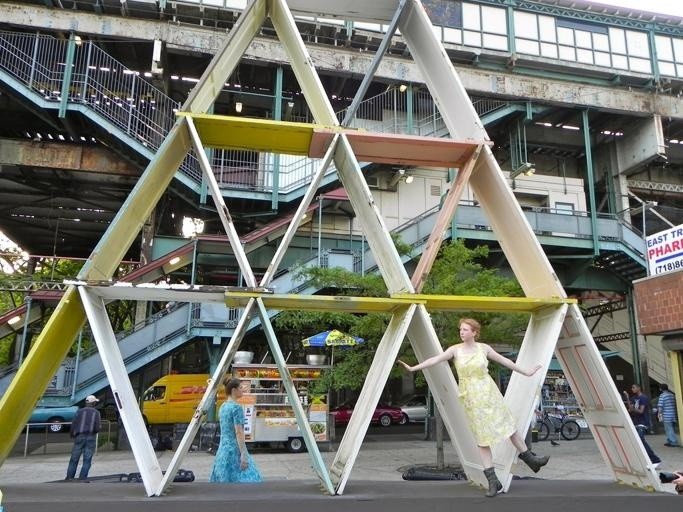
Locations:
[283,396,308,405]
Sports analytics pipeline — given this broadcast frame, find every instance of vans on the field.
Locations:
[395,393,426,426]
[134,374,229,426]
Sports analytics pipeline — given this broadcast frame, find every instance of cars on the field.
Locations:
[650,397,663,429]
[330,397,403,427]
[25,406,80,432]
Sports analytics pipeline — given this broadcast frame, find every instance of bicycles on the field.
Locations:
[535,409,580,441]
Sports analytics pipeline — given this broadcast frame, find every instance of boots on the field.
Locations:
[518,449,550,473]
[483,467,502,497]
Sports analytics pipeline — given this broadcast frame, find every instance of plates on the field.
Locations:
[308,422,325,435]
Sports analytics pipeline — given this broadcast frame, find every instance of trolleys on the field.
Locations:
[230,363,332,452]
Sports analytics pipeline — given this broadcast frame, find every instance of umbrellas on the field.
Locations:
[301,329,365,377]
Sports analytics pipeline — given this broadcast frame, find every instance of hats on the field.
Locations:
[86,395,99,403]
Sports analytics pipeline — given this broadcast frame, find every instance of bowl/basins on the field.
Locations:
[305,354,326,366]
[232,351,254,363]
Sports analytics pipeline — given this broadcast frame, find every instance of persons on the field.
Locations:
[396,316,551,498]
[65,395,102,481]
[156,387,165,399]
[210,376,262,484]
[657,384,679,448]
[672,473,683,492]
[624,382,661,468]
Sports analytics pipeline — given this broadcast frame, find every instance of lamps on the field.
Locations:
[390,170,414,188]
